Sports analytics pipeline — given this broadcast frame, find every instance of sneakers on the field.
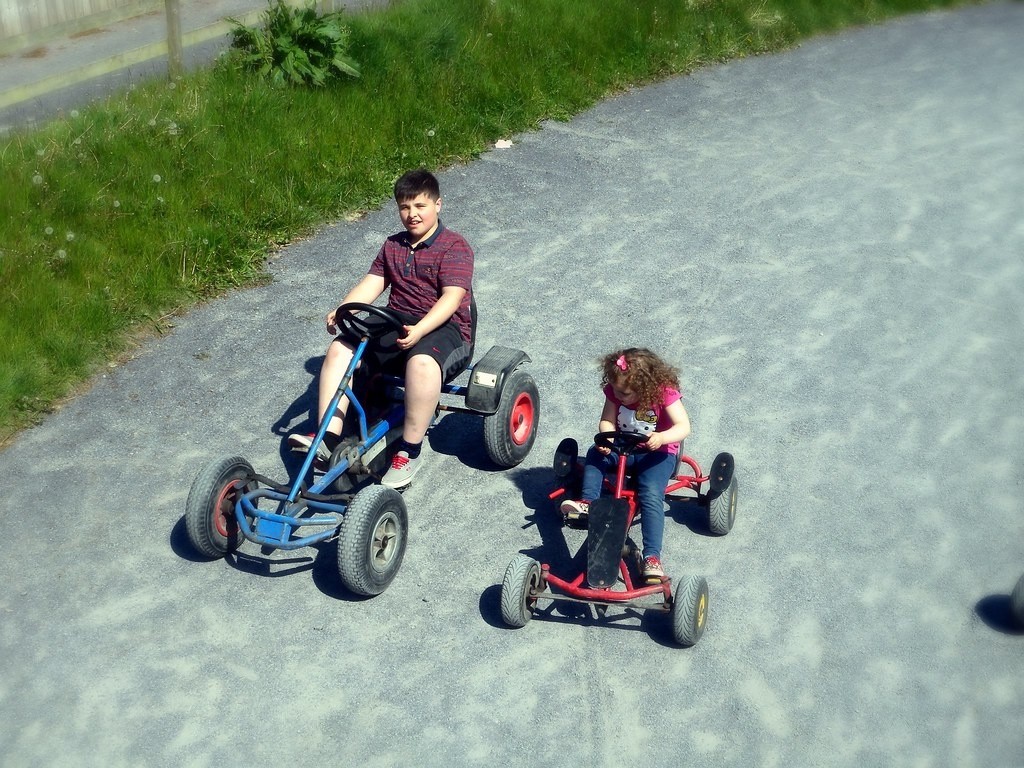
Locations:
[288,432,333,460]
[642,554,665,579]
[559,498,592,517]
[380,451,423,487]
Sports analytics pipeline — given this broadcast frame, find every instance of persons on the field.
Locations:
[561,348,690,584]
[287,167,474,488]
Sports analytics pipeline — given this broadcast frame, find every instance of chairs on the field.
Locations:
[671,440,684,476]
[384,287,477,385]
